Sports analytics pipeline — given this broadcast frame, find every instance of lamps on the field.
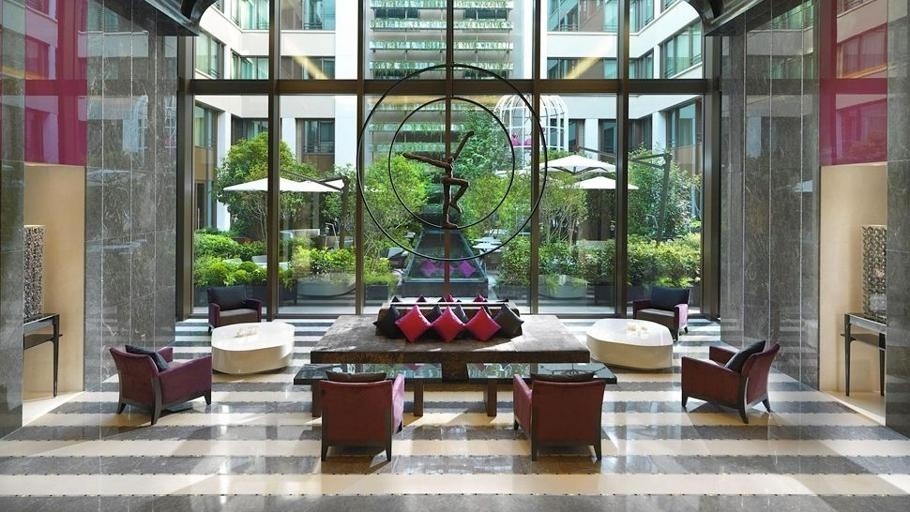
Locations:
[24,225,46,321]
[862,224,887,324]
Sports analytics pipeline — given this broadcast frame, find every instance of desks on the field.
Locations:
[538,275,587,298]
[311,314,590,381]
[24,313,63,397]
[297,272,356,296]
[840,312,887,396]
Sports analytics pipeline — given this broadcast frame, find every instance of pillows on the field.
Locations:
[211,287,247,310]
[649,287,690,311]
[418,256,477,279]
[124,344,172,373]
[724,340,766,373]
[531,371,595,382]
[372,293,525,343]
[325,370,387,382]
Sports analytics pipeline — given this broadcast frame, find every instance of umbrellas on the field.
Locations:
[222,177,381,193]
[495,143,673,246]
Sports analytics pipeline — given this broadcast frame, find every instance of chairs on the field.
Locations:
[681,344,781,424]
[513,374,607,461]
[207,287,264,333]
[319,373,405,461]
[109,347,212,425]
[633,298,688,341]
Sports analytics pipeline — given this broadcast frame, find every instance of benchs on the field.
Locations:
[464,362,617,416]
[293,362,442,417]
